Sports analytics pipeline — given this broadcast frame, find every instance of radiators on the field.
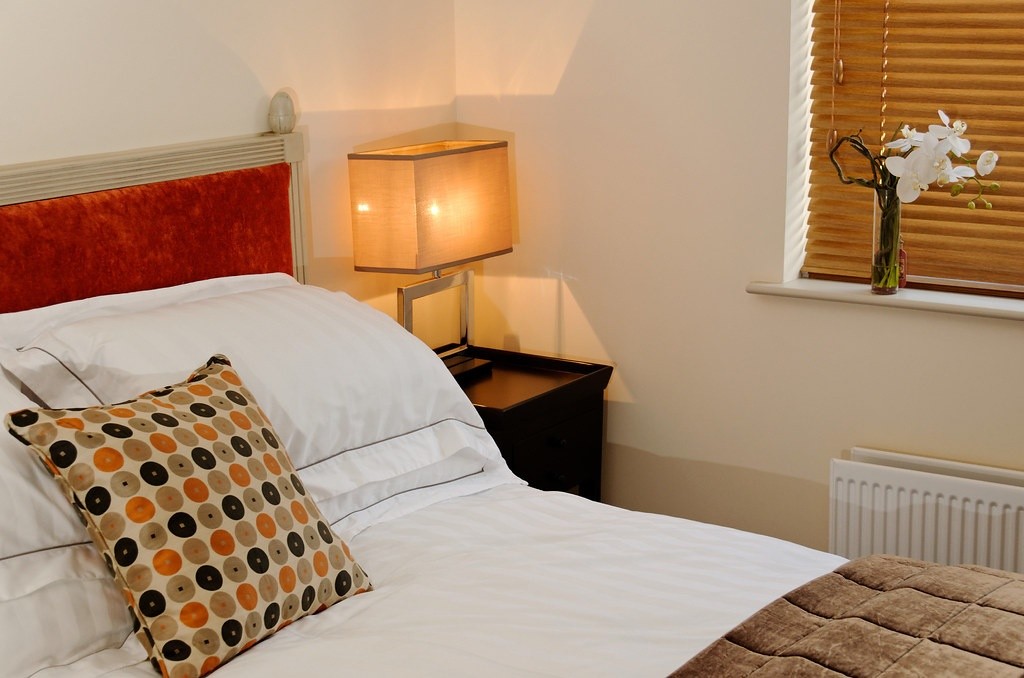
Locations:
[827,446,1023,574]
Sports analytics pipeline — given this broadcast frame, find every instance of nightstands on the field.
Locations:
[449,349,614,500]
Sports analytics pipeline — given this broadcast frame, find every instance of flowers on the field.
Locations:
[828,106,998,212]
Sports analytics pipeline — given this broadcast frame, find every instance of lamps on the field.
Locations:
[348,137,512,385]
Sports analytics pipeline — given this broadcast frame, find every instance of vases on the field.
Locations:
[870,186,906,294]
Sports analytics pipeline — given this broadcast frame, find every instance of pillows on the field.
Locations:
[5,355,374,677]
[1,275,502,603]
[0,448,530,678]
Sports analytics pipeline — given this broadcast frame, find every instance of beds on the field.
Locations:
[1,88,1024,678]
